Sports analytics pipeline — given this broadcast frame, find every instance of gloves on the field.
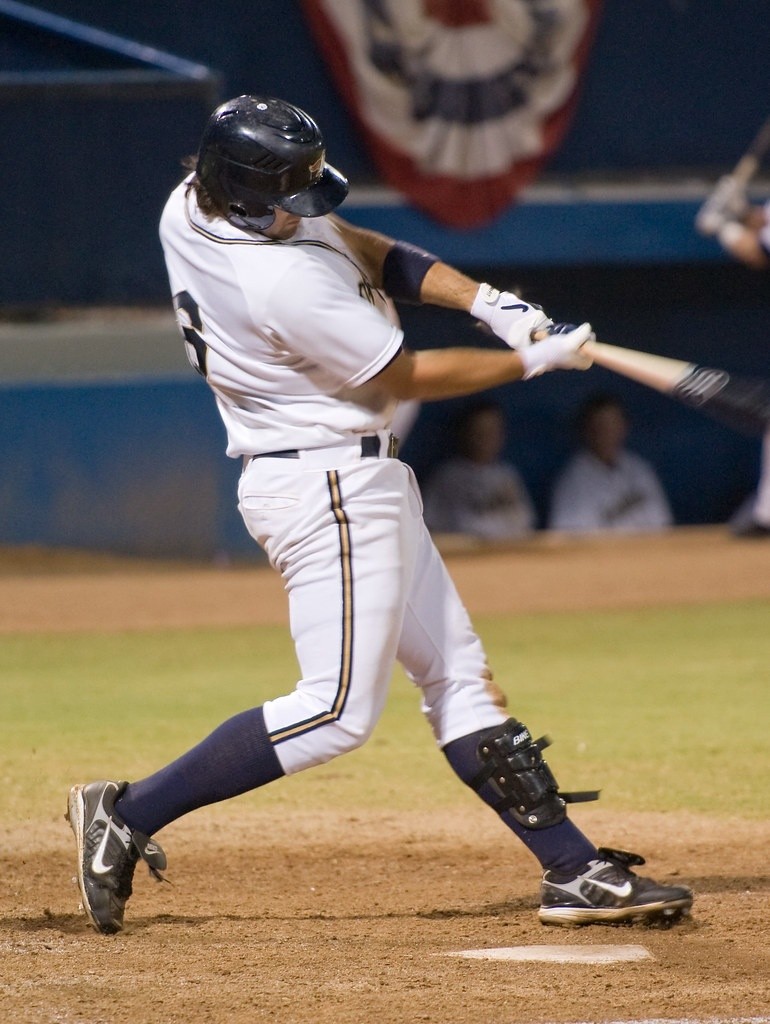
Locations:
[471,280,553,351]
[514,321,597,382]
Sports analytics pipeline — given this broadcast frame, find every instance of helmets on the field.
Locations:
[194,95,350,231]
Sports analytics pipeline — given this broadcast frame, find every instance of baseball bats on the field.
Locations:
[535,319,769,440]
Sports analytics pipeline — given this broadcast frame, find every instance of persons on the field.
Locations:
[419,128,770,538]
[65,95,693,934]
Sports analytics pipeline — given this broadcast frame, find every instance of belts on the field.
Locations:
[252,434,398,459]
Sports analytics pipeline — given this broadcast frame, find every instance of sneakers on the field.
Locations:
[63,779,175,936]
[538,847,694,928]
